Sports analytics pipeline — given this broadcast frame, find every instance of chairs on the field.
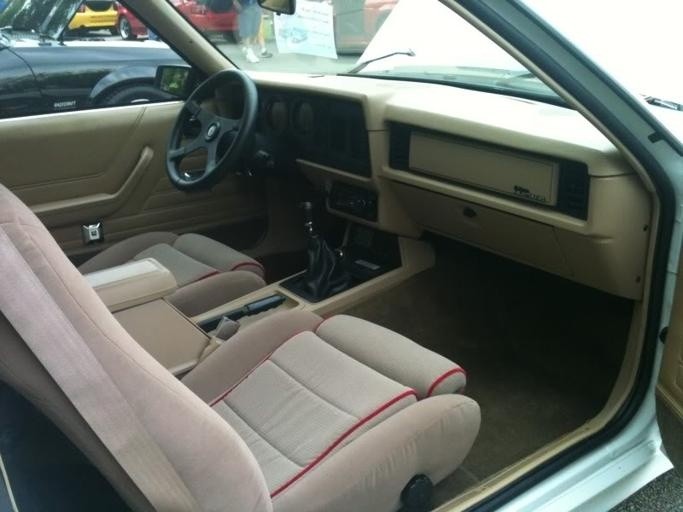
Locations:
[0,184,481,512]
[79,231,266,318]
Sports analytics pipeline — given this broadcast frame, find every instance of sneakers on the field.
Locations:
[259,52,273,57]
[246,54,260,63]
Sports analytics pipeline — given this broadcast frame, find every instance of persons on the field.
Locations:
[232,0,262,65]
[256,12,273,58]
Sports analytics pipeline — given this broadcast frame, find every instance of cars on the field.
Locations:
[63,0,119,37]
[0,0,683,512]
[114,0,240,44]
[0,0,190,119]
[362,0,399,41]
